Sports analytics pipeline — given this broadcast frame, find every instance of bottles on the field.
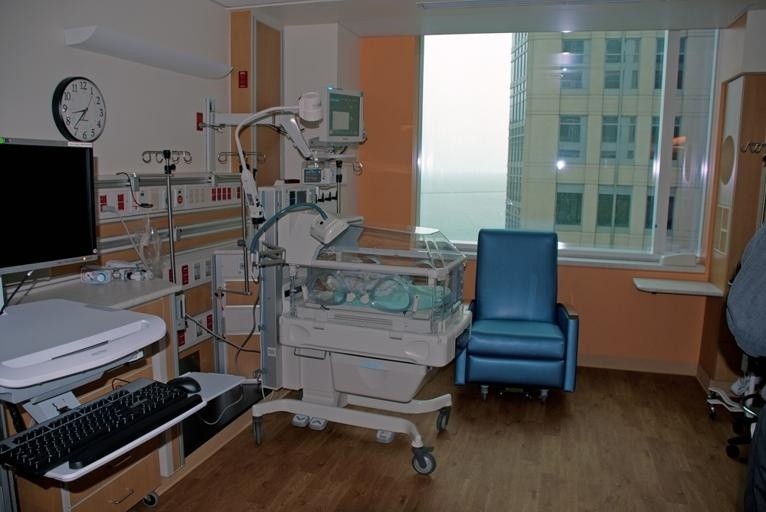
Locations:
[79,258,154,285]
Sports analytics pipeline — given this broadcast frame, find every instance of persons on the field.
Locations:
[725,222,766,512]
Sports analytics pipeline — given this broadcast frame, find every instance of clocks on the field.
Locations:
[50,74,107,144]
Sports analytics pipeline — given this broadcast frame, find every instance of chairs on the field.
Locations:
[452,229,581,404]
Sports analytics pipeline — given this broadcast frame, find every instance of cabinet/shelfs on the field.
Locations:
[0,296,183,512]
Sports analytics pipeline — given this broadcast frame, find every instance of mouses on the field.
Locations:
[165,377,201,393]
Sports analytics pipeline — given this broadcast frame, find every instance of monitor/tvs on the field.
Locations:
[319,88,364,143]
[0,136,98,276]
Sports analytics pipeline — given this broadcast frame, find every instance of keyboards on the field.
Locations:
[0,376,189,479]
